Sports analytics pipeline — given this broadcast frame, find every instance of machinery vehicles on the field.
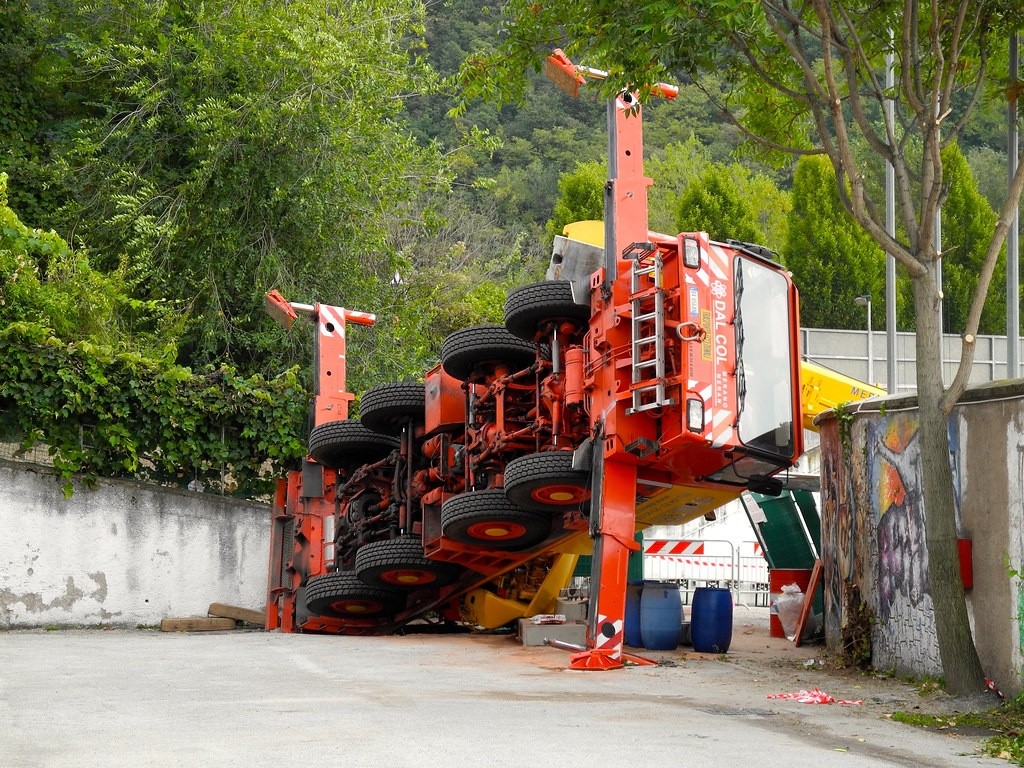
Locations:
[256,44,889,674]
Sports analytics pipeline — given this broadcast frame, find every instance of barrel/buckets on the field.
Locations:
[768,569,813,639]
[625,579,682,650]
[691,587,733,653]
